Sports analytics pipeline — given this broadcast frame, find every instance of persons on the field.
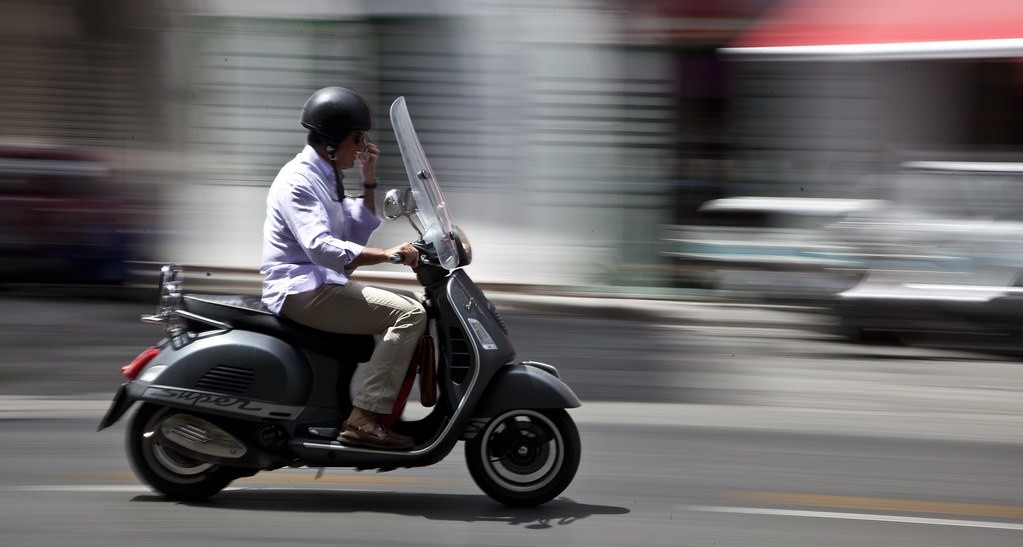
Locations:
[258,86,426,454]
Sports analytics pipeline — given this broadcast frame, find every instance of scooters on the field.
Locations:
[97,94,585,507]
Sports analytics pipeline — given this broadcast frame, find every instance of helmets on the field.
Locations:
[300,86,374,159]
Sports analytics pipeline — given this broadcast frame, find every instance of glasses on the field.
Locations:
[345,131,365,147]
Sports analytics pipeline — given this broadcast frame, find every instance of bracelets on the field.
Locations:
[362,182,378,189]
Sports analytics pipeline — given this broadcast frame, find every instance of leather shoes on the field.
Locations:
[338,419,415,450]
